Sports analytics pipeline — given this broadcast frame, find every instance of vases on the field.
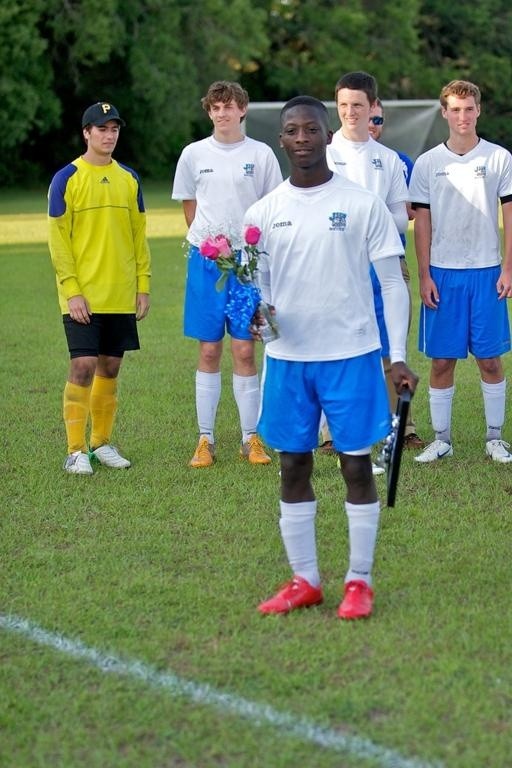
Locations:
[252,301,278,343]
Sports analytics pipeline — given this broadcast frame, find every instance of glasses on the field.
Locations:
[369,117,385,124]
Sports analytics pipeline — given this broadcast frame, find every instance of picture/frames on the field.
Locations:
[387,390,411,508]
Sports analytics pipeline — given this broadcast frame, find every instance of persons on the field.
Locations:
[315,72,412,476]
[47,101,151,476]
[171,80,284,469]
[240,95,421,620]
[319,98,424,454]
[409,80,512,463]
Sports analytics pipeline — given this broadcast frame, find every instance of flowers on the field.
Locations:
[180,222,269,332]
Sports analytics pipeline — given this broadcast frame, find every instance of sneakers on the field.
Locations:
[64,451,93,474]
[240,436,271,464]
[338,581,373,620]
[89,444,131,469]
[414,440,453,463]
[321,441,337,455]
[337,456,385,476]
[191,438,215,467]
[257,577,324,612]
[404,434,424,449]
[484,440,512,464]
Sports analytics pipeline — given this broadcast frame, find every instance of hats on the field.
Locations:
[82,102,125,127]
